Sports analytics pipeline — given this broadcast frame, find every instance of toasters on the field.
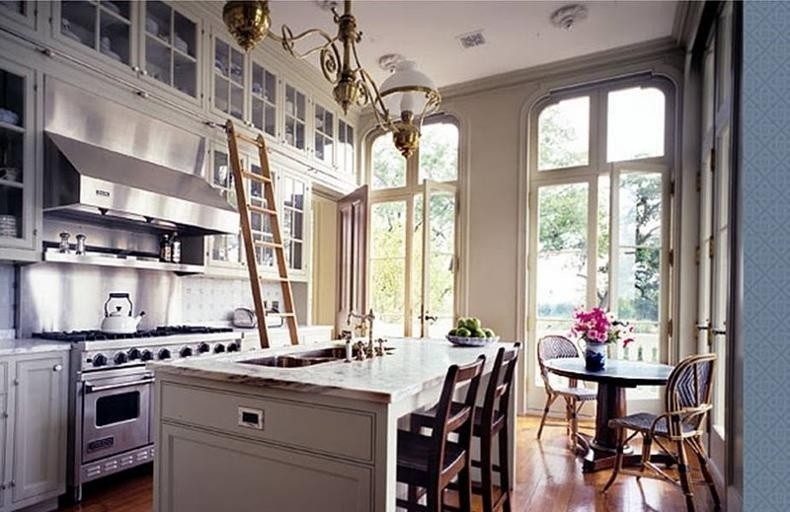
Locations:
[232,306,284,328]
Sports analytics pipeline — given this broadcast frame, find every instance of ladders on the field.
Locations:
[226,119,300,349]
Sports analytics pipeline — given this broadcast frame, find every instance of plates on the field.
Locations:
[446,334,500,347]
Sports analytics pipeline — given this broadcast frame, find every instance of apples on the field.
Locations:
[448,318,494,337]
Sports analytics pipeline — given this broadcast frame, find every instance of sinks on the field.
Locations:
[292,347,378,359]
[237,356,327,368]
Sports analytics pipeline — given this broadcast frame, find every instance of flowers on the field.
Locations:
[569,306,638,352]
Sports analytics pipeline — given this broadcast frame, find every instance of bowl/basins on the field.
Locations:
[0,109,19,124]
[146,16,160,35]
[174,37,188,54]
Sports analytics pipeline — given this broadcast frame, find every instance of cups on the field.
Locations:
[0,214,18,237]
[286,99,294,115]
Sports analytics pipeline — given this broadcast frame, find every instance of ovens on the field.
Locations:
[69,366,156,504]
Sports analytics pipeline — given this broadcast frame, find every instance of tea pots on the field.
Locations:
[101,292,145,334]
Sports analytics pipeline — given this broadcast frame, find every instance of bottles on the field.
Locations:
[75,235,87,256]
[161,231,183,263]
[59,232,70,254]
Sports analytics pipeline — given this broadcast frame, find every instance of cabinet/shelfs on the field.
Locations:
[1,1,44,44]
[0,57,41,254]
[1,336,71,510]
[44,0,210,101]
[336,106,362,186]
[208,29,282,144]
[238,330,305,354]
[207,155,310,279]
[282,65,336,173]
[306,328,332,346]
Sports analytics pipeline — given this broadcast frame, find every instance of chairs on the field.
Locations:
[406,340,527,512]
[395,354,487,512]
[597,355,723,511]
[537,335,602,451]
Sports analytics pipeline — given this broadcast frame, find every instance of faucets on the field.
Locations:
[347,310,375,357]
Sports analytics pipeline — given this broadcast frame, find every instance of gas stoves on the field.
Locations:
[31,323,245,373]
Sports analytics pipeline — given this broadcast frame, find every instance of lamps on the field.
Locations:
[216,0,441,158]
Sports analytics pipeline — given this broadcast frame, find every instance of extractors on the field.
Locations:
[43,73,242,238]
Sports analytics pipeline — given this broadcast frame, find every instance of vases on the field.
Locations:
[585,350,604,370]
